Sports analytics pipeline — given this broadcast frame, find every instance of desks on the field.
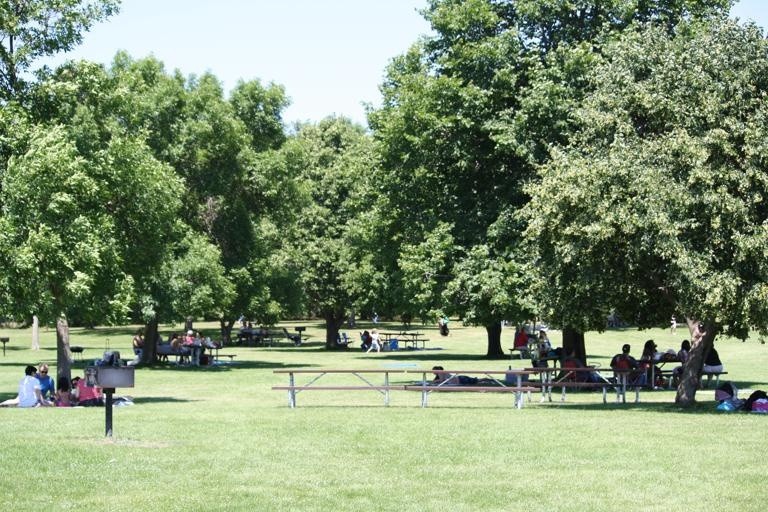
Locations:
[523,367,635,402]
[274,370,538,407]
[635,359,683,390]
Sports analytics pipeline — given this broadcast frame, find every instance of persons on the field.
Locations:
[1,363,130,407]
[669,313,677,335]
[335,328,381,354]
[430,366,505,386]
[132,327,221,362]
[371,312,377,325]
[235,321,274,346]
[437,315,452,337]
[512,325,723,389]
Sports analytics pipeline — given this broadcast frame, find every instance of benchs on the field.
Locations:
[377,333,430,350]
[270,387,540,410]
[658,370,728,389]
[520,382,625,409]
[155,347,237,368]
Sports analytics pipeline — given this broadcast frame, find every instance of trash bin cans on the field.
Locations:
[391,339,398,351]
